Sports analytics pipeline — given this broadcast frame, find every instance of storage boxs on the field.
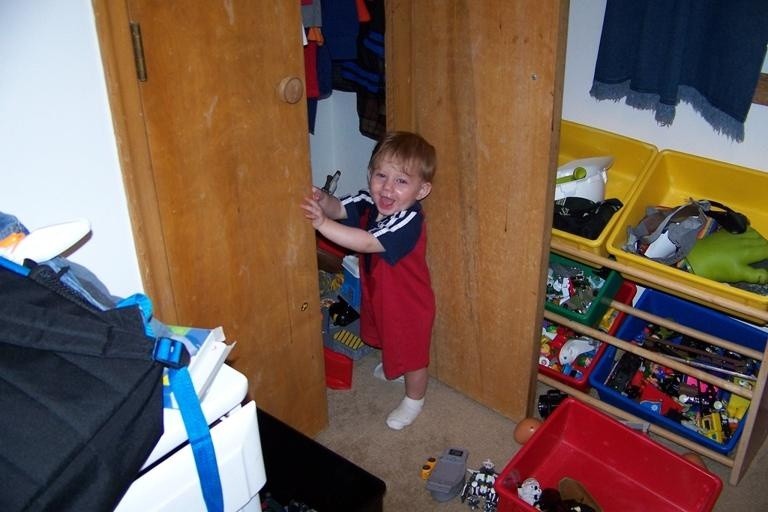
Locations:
[492,120,768,485]
[255,406,388,512]
[494,396,722,512]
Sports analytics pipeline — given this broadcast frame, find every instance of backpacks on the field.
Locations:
[0,257,226,512]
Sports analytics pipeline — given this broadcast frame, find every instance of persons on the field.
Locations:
[297,130,439,433]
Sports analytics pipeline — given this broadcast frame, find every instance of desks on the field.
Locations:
[113,361,268,512]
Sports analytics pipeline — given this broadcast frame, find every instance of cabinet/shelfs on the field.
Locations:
[90,0,572,438]
